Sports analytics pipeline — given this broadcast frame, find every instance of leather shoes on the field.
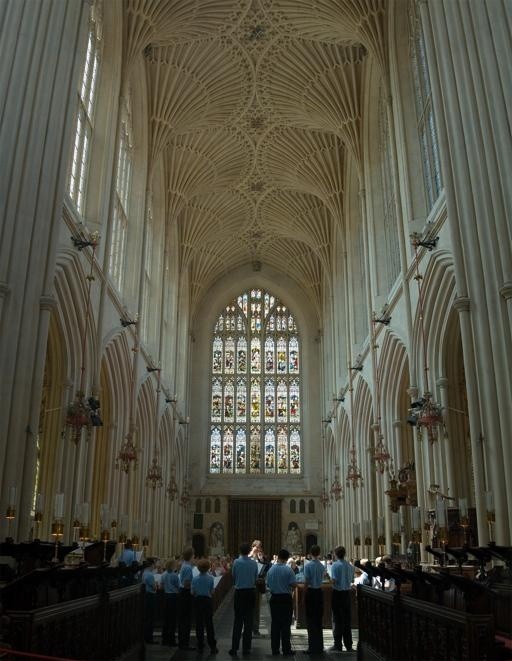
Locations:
[144,639,353,657]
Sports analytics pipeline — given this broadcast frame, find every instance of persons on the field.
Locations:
[119,539,395,656]
